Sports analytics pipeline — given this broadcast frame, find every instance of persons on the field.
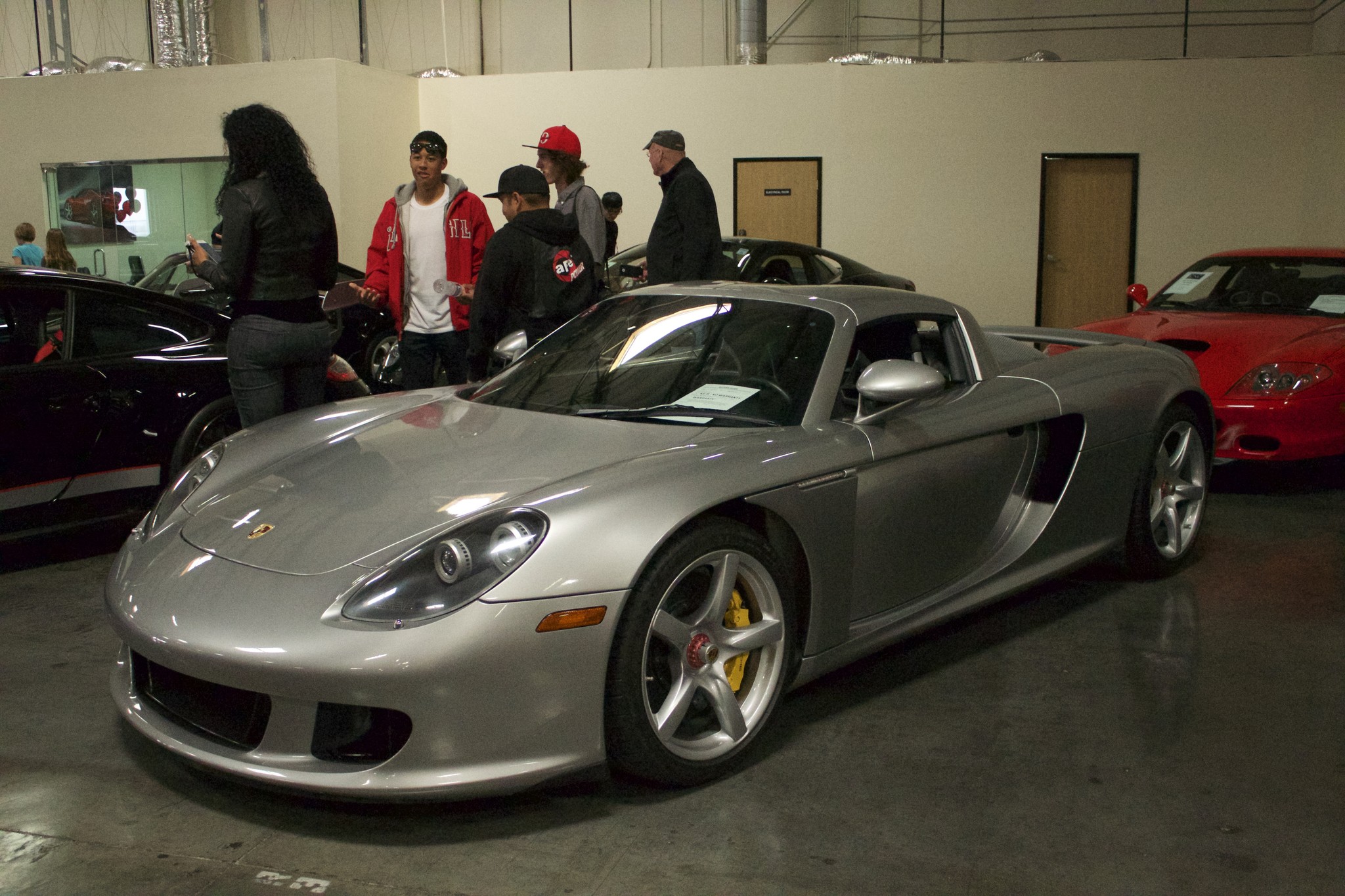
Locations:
[347,129,495,392]
[601,192,623,262]
[643,129,723,290]
[41,227,77,273]
[183,104,339,433]
[463,164,598,385]
[11,223,44,269]
[521,125,608,293]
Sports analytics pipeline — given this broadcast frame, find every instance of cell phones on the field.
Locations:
[186,240,220,264]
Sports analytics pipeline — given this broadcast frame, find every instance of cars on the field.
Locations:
[0,263,372,539]
[488,236,918,390]
[132,248,451,391]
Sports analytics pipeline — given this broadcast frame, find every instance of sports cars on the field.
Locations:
[1043,247,1345,468]
[102,279,1221,806]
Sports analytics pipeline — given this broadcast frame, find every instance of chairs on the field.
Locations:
[1221,264,1273,307]
[756,260,795,284]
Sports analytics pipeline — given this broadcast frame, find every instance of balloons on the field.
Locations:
[112,187,141,223]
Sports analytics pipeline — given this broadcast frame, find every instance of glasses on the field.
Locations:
[409,141,443,155]
[647,148,660,157]
[607,207,623,216]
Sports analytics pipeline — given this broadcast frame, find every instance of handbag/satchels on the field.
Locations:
[563,187,618,261]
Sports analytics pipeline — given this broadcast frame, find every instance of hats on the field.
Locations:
[522,124,581,160]
[642,130,686,151]
[483,165,548,199]
